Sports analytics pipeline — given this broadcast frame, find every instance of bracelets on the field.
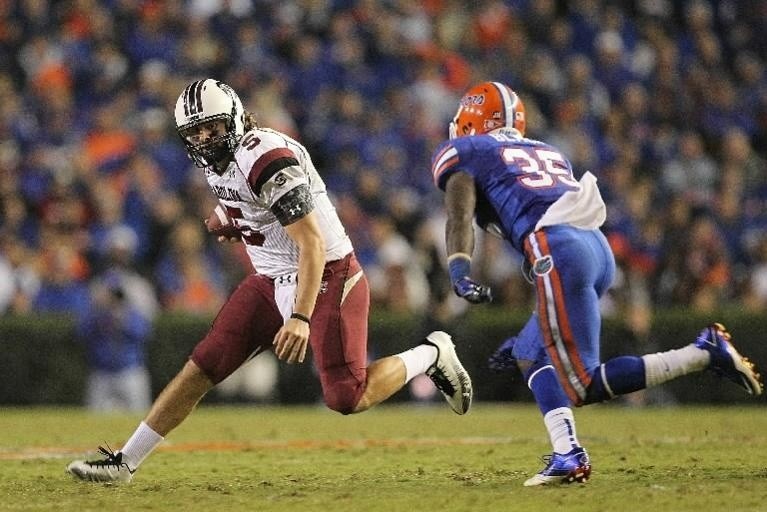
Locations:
[290,313,310,326]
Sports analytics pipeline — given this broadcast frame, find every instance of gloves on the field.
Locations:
[446,254,494,304]
[485,336,519,373]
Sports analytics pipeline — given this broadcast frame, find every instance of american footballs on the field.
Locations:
[207,202,241,238]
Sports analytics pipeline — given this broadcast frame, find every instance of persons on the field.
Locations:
[0,0,767,316]
[432,79,762,486]
[598,234,652,408]
[64,76,474,488]
[68,224,159,413]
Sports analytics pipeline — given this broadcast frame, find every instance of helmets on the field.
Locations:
[174,77,245,155]
[447,80,527,142]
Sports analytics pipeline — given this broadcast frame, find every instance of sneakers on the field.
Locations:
[694,322,763,397]
[419,328,473,416]
[524,445,593,488]
[67,449,135,487]
